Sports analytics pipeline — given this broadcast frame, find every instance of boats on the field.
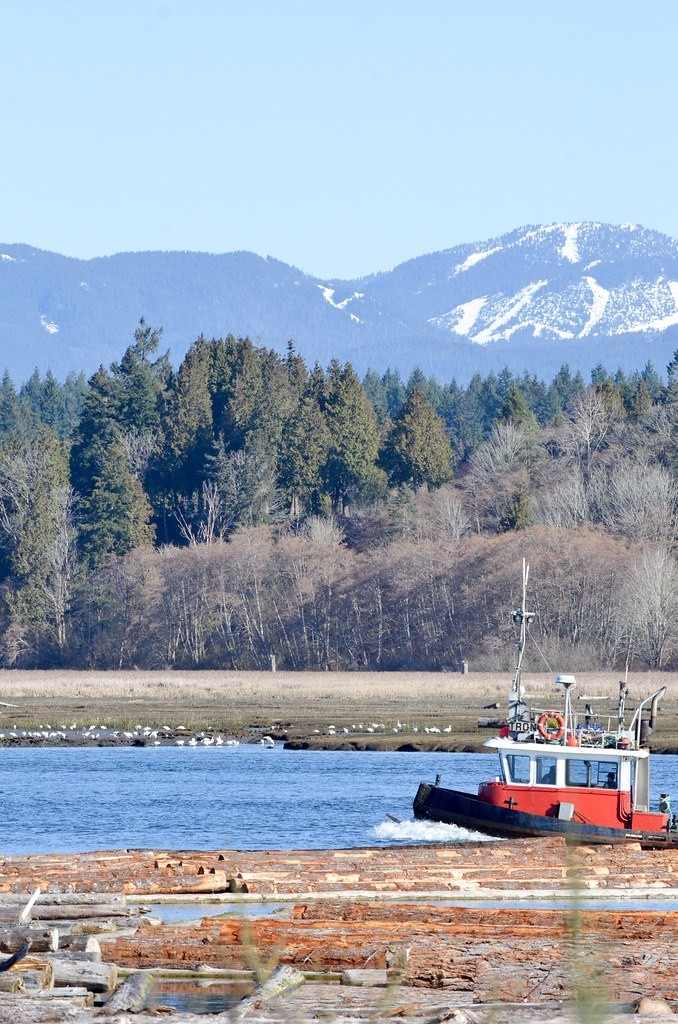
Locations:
[386,557,678,848]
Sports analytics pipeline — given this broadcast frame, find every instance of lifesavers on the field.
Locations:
[538,712,565,740]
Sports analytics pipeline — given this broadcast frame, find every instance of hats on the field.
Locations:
[605,773,615,778]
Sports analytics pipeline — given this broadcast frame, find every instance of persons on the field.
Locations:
[500,725,510,738]
[602,772,617,789]
[541,766,556,784]
